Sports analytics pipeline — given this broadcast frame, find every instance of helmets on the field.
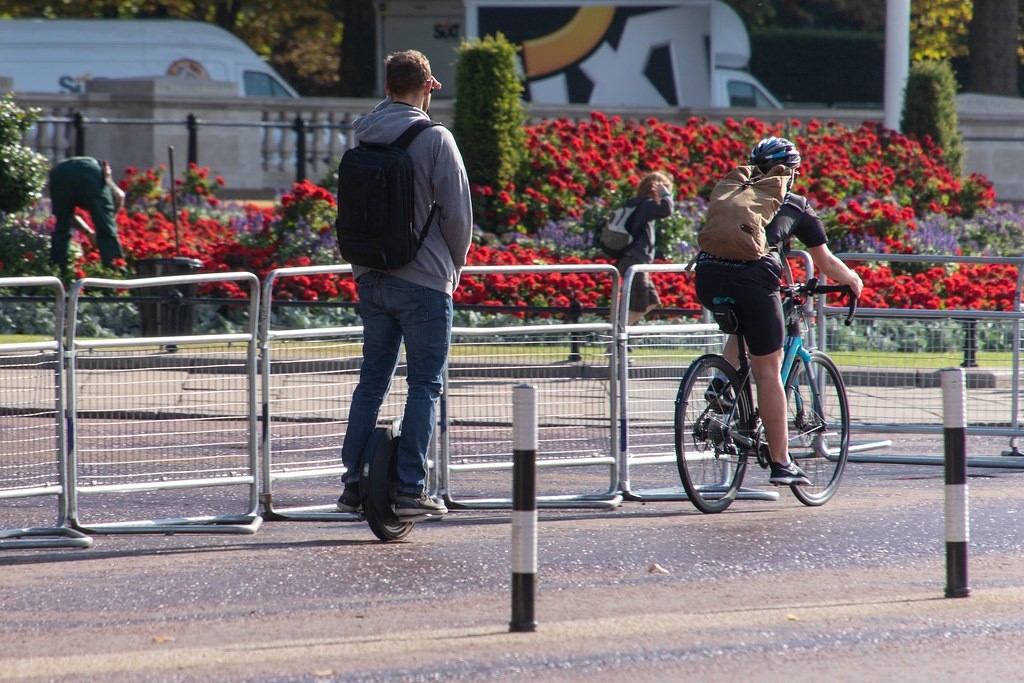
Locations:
[747,136,801,169]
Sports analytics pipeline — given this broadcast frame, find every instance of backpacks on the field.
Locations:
[600,195,656,258]
[683,165,796,272]
[335,120,445,269]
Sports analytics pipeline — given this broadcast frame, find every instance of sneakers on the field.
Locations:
[769,452,812,485]
[704,378,735,408]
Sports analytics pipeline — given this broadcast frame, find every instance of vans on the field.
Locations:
[0,15,350,199]
[372,0,800,141]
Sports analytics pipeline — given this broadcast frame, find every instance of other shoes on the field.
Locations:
[337,483,362,512]
[608,357,632,367]
[396,494,449,516]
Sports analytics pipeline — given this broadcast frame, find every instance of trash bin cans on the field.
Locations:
[132,257,204,337]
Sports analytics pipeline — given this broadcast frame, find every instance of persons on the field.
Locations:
[605,171,674,366]
[41,157,125,276]
[337,48,472,515]
[695,136,863,487]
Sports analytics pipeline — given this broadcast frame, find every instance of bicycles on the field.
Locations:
[674,276,856,515]
